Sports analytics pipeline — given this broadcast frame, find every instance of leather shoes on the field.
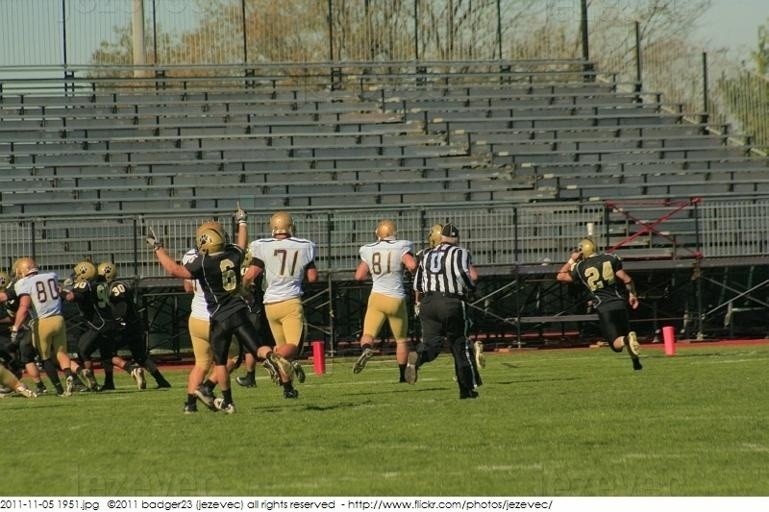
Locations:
[233,201,247,223]
[146,227,160,252]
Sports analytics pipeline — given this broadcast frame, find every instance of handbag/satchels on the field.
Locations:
[629,291,637,297]
[568,258,575,265]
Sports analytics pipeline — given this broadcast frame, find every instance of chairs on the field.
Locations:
[184,353,305,413]
[354,341,485,398]
[0,368,172,397]
[627,331,641,356]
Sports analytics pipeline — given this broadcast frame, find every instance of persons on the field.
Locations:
[404,224,482,399]
[0,257,171,398]
[182,247,243,412]
[557,239,642,370]
[239,212,318,398]
[353,220,418,383]
[146,201,294,413]
[235,225,277,387]
[414,224,486,370]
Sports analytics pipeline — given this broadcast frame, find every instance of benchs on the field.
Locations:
[1,61,769,253]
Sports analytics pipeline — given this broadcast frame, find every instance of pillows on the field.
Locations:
[375,220,397,240]
[196,221,226,254]
[427,224,443,247]
[270,211,293,237]
[0,257,117,290]
[577,239,596,259]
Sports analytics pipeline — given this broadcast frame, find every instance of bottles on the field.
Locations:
[441,225,458,237]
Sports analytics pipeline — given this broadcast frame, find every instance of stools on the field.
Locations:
[422,292,462,299]
